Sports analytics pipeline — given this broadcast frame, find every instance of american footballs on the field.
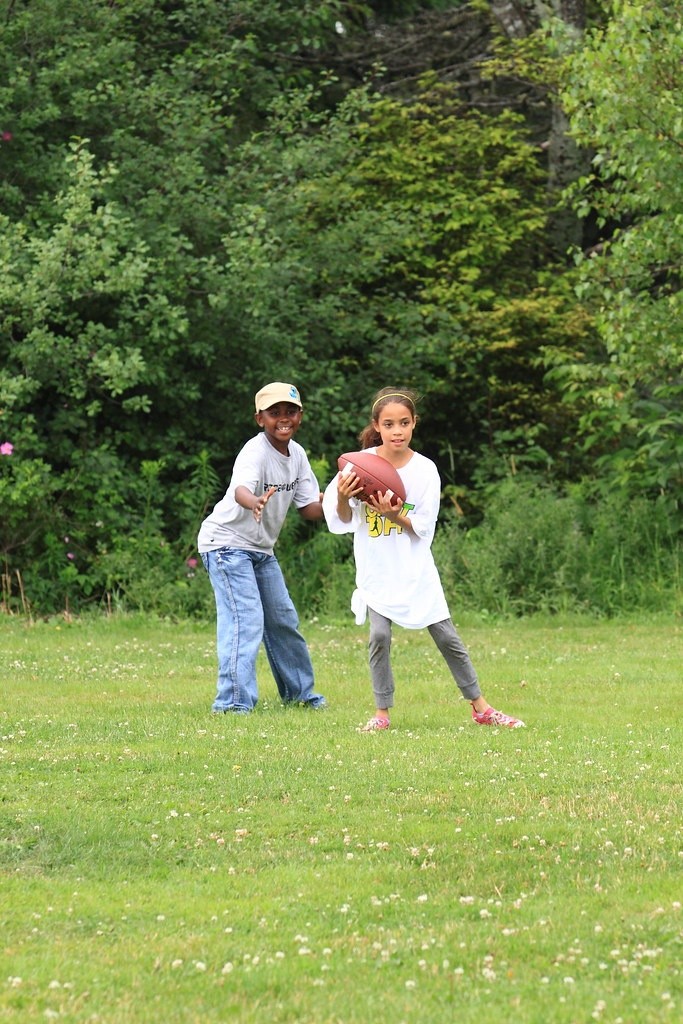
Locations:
[338,452,407,508]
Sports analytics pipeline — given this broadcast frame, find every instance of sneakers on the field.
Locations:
[358,717,389,734]
[472,703,524,730]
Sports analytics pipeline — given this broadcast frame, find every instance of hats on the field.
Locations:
[255,382,302,413]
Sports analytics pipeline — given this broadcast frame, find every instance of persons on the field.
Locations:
[197,381,331,718]
[322,385,528,735]
